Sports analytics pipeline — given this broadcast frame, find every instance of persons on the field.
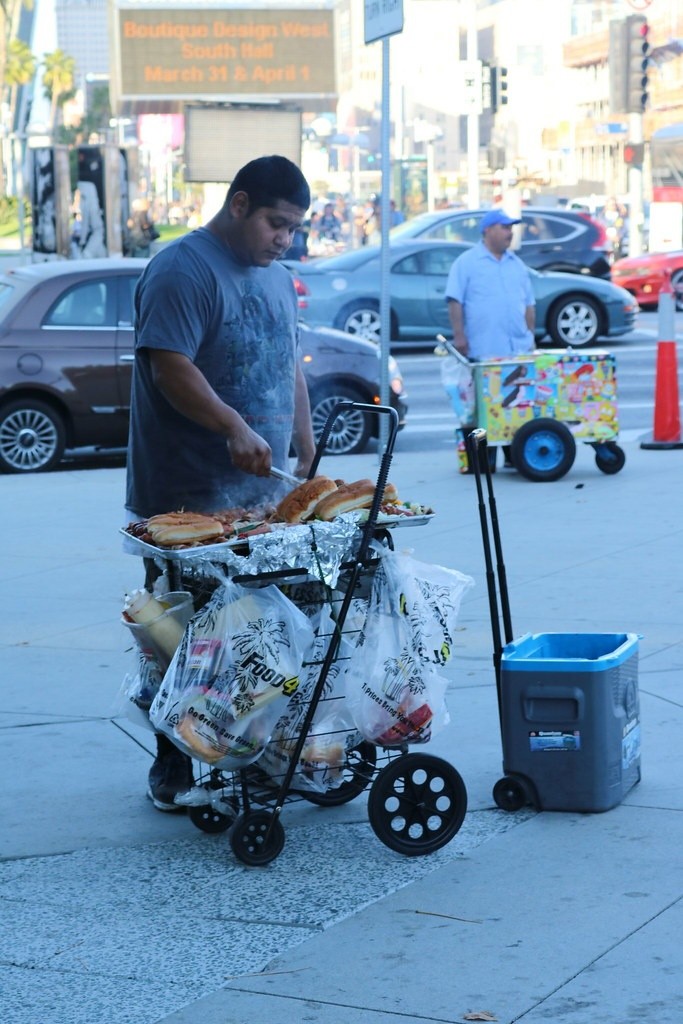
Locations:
[124,197,405,260]
[127,156,315,810]
[599,198,626,262]
[445,210,535,468]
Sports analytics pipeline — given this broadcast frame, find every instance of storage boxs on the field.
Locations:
[499,627,640,813]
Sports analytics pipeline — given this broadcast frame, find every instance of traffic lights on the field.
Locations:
[623,16,650,115]
[491,65,509,112]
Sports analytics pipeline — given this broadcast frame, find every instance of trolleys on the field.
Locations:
[436,337,625,482]
[121,400,467,862]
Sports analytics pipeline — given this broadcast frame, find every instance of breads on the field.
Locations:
[144,476,399,546]
[304,742,342,782]
[175,712,228,761]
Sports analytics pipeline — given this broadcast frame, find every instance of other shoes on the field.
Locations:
[504,460,512,467]
[147,759,193,812]
[480,463,495,474]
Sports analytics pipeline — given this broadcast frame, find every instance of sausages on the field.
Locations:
[387,507,415,516]
[128,509,270,551]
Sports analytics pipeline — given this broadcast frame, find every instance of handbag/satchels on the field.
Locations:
[110,651,170,734]
[441,357,476,426]
[147,549,476,794]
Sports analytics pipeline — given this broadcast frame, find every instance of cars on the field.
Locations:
[0,258,411,471]
[285,238,641,353]
[610,245,683,310]
[390,203,610,279]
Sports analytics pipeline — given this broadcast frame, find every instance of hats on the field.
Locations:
[479,209,522,233]
